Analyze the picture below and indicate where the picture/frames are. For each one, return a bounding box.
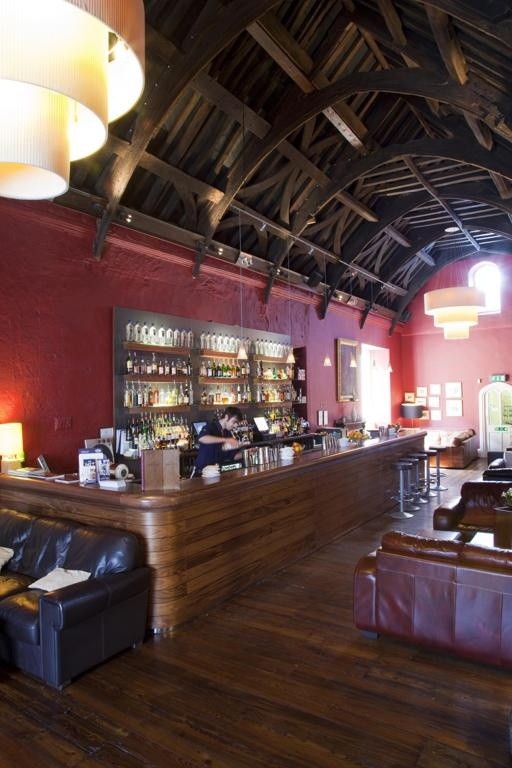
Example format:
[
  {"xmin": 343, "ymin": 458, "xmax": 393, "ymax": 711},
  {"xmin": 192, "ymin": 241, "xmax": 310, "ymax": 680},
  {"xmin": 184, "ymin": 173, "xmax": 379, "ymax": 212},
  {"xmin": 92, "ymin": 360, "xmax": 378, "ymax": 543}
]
[
  {"xmin": 336, "ymin": 339, "xmax": 361, "ymax": 403},
  {"xmin": 404, "ymin": 392, "xmax": 415, "ymax": 403}
]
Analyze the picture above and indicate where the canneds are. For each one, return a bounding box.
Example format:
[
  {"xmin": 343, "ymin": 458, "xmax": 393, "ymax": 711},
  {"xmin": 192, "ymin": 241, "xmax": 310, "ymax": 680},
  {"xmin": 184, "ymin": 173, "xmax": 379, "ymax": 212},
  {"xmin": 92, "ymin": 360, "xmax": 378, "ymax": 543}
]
[
  {"xmin": 243, "ymin": 447, "xmax": 278, "ymax": 468},
  {"xmin": 322, "ymin": 436, "xmax": 339, "ymax": 450}
]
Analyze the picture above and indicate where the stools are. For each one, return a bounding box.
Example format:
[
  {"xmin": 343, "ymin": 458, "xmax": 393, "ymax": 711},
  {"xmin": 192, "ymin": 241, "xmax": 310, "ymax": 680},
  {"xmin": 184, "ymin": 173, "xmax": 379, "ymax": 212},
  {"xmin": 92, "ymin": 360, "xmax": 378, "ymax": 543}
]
[
  {"xmin": 407, "ymin": 453, "xmax": 428, "ymax": 504},
  {"xmin": 429, "ymin": 446, "xmax": 448, "ymax": 491},
  {"xmin": 419, "ymin": 450, "xmax": 438, "ymax": 497},
  {"xmin": 399, "ymin": 458, "xmax": 420, "ymax": 512},
  {"xmin": 390, "ymin": 461, "xmax": 414, "ymax": 520}
]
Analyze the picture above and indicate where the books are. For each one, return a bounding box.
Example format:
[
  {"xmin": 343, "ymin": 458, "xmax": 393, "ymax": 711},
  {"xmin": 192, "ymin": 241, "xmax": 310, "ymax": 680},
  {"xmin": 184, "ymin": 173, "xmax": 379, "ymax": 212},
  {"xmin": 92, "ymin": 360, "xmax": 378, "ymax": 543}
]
[{"xmin": 11, "ymin": 467, "xmax": 81, "ymax": 484}]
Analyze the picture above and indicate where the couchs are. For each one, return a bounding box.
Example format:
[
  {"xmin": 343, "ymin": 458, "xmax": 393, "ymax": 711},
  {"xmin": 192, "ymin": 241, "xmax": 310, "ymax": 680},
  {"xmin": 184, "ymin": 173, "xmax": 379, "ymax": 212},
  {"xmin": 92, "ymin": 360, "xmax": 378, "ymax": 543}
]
[
  {"xmin": 401, "ymin": 428, "xmax": 480, "ymax": 469},
  {"xmin": 0, "ymin": 504, "xmax": 155, "ymax": 688},
  {"xmin": 483, "ymin": 448, "xmax": 512, "ymax": 481},
  {"xmin": 433, "ymin": 480, "xmax": 512, "ymax": 531},
  {"xmin": 355, "ymin": 530, "xmax": 512, "ymax": 671}
]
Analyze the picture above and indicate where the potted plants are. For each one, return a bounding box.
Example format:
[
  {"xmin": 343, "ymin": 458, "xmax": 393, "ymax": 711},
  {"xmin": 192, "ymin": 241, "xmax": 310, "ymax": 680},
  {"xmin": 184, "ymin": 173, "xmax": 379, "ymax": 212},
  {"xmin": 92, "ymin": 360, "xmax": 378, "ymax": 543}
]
[{"xmin": 491, "ymin": 488, "xmax": 512, "ymax": 531}]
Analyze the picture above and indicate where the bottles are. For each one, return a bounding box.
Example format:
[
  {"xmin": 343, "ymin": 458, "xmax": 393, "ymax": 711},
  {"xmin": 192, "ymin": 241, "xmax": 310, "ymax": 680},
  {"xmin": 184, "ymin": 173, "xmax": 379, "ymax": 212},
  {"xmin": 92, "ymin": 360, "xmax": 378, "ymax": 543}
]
[
  {"xmin": 222, "ymin": 383, "xmax": 252, "ymax": 403},
  {"xmin": 166, "ymin": 384, "xmax": 172, "ymax": 405},
  {"xmin": 146, "ymin": 359, "xmax": 152, "ymax": 373},
  {"xmin": 158, "ymin": 359, "xmax": 164, "ymax": 374},
  {"xmin": 223, "ymin": 333, "xmax": 230, "ymax": 351},
  {"xmin": 217, "ymin": 332, "xmax": 224, "ymax": 351},
  {"xmin": 200, "ymin": 330, "xmax": 206, "ymax": 349},
  {"xmin": 240, "ymin": 361, "xmax": 246, "ymax": 377},
  {"xmin": 138, "ymin": 384, "xmax": 143, "ymax": 406},
  {"xmin": 215, "ymin": 384, "xmax": 222, "ymax": 404},
  {"xmin": 171, "ymin": 354, "xmax": 191, "ymax": 374},
  {"xmin": 140, "ymin": 353, "xmax": 146, "ymax": 374},
  {"xmin": 164, "ymin": 358, "xmax": 169, "ymax": 374},
  {"xmin": 199, "ymin": 360, "xmax": 207, "ymax": 377},
  {"xmin": 150, "ymin": 322, "xmax": 157, "ymax": 344},
  {"xmin": 236, "ymin": 335, "xmax": 251, "ymax": 353},
  {"xmin": 260, "ymin": 338, "xmax": 264, "ymax": 354},
  {"xmin": 125, "ymin": 319, "xmax": 134, "ymax": 341},
  {"xmin": 178, "ymin": 383, "xmax": 184, "ymax": 405},
  {"xmin": 210, "ymin": 331, "xmax": 217, "ymax": 349},
  {"xmin": 180, "ymin": 327, "xmax": 188, "ymax": 347},
  {"xmin": 255, "ymin": 383, "xmax": 292, "ymax": 402},
  {"xmin": 264, "ymin": 339, "xmax": 293, "ymax": 357},
  {"xmin": 255, "ymin": 338, "xmax": 260, "ymax": 354},
  {"xmin": 207, "ymin": 360, "xmax": 212, "ymax": 376},
  {"xmin": 148, "ymin": 384, "xmax": 154, "ymax": 406},
  {"xmin": 172, "ymin": 383, "xmax": 178, "ymax": 405},
  {"xmin": 206, "ymin": 331, "xmax": 211, "ymax": 349},
  {"xmin": 133, "ymin": 351, "xmax": 139, "ymax": 373},
  {"xmin": 142, "ymin": 321, "xmax": 150, "ymax": 344},
  {"xmin": 201, "ymin": 386, "xmax": 207, "ymax": 405},
  {"xmin": 166, "ymin": 325, "xmax": 173, "ymax": 346},
  {"xmin": 152, "ymin": 352, "xmax": 157, "ymax": 373},
  {"xmin": 187, "ymin": 327, "xmax": 194, "ymax": 347},
  {"xmin": 154, "ymin": 384, "xmax": 159, "ymax": 406},
  {"xmin": 222, "ymin": 441, "xmax": 251, "ymax": 451},
  {"xmin": 126, "ymin": 412, "xmax": 194, "ymax": 450},
  {"xmin": 125, "ymin": 349, "xmax": 133, "ymax": 373},
  {"xmin": 246, "ymin": 362, "xmax": 251, "ymax": 377},
  {"xmin": 130, "ymin": 384, "xmax": 137, "ymax": 407},
  {"xmin": 143, "ymin": 384, "xmax": 148, "ymax": 407},
  {"xmin": 134, "ymin": 320, "xmax": 142, "ymax": 342},
  {"xmin": 173, "ymin": 327, "xmax": 181, "ymax": 347},
  {"xmin": 229, "ymin": 334, "xmax": 236, "ymax": 352},
  {"xmin": 184, "ymin": 383, "xmax": 189, "ymax": 404},
  {"xmin": 123, "ymin": 383, "xmax": 130, "ymax": 407},
  {"xmin": 264, "ymin": 408, "xmax": 304, "ymax": 436},
  {"xmin": 256, "ymin": 360, "xmax": 293, "ymax": 380},
  {"xmin": 159, "ymin": 387, "xmax": 166, "ymax": 404},
  {"xmin": 157, "ymin": 323, "xmax": 166, "ymax": 345},
  {"xmin": 207, "ymin": 387, "xmax": 214, "ymax": 405},
  {"xmin": 222, "ymin": 361, "xmax": 241, "ymax": 377},
  {"xmin": 215, "ymin": 408, "xmax": 225, "ymax": 418},
  {"xmin": 213, "ymin": 360, "xmax": 222, "ymax": 377}
]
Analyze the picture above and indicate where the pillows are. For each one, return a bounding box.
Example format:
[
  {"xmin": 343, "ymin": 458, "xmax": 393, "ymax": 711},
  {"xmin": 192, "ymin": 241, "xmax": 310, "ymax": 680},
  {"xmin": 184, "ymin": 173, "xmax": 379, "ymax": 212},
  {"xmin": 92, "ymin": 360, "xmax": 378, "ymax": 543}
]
[
  {"xmin": 0, "ymin": 547, "xmax": 14, "ymax": 571},
  {"xmin": 28, "ymin": 567, "xmax": 91, "ymax": 592}
]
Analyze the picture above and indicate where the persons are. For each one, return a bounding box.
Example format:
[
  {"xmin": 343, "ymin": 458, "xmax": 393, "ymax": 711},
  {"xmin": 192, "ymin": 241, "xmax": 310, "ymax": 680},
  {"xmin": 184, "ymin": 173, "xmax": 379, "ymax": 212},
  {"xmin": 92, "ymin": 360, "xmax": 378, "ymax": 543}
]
[{"xmin": 195, "ymin": 406, "xmax": 243, "ymax": 477}]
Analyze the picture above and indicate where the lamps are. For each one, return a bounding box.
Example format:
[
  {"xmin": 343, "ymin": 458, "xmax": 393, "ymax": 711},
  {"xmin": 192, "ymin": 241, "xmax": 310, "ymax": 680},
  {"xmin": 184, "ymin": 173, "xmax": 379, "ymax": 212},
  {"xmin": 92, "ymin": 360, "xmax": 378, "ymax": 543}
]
[
  {"xmin": 0, "ymin": 0, "xmax": 146, "ymax": 200},
  {"xmin": 0, "ymin": 422, "xmax": 23, "ymax": 474},
  {"xmin": 322, "ymin": 253, "xmax": 333, "ymax": 367},
  {"xmin": 349, "ymin": 274, "xmax": 357, "ymax": 368},
  {"xmin": 285, "ymin": 241, "xmax": 296, "ymax": 364},
  {"xmin": 235, "ymin": 208, "xmax": 249, "ymax": 360},
  {"xmin": 401, "ymin": 403, "xmax": 422, "ymax": 428},
  {"xmin": 424, "ymin": 227, "xmax": 486, "ymax": 340}
]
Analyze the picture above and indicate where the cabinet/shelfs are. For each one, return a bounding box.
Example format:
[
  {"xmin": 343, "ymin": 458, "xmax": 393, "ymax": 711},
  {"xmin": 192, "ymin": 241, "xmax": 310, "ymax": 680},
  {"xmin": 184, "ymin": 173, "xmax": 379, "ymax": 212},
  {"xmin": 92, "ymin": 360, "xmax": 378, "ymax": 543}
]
[{"xmin": 112, "ymin": 306, "xmax": 295, "ymax": 476}]
